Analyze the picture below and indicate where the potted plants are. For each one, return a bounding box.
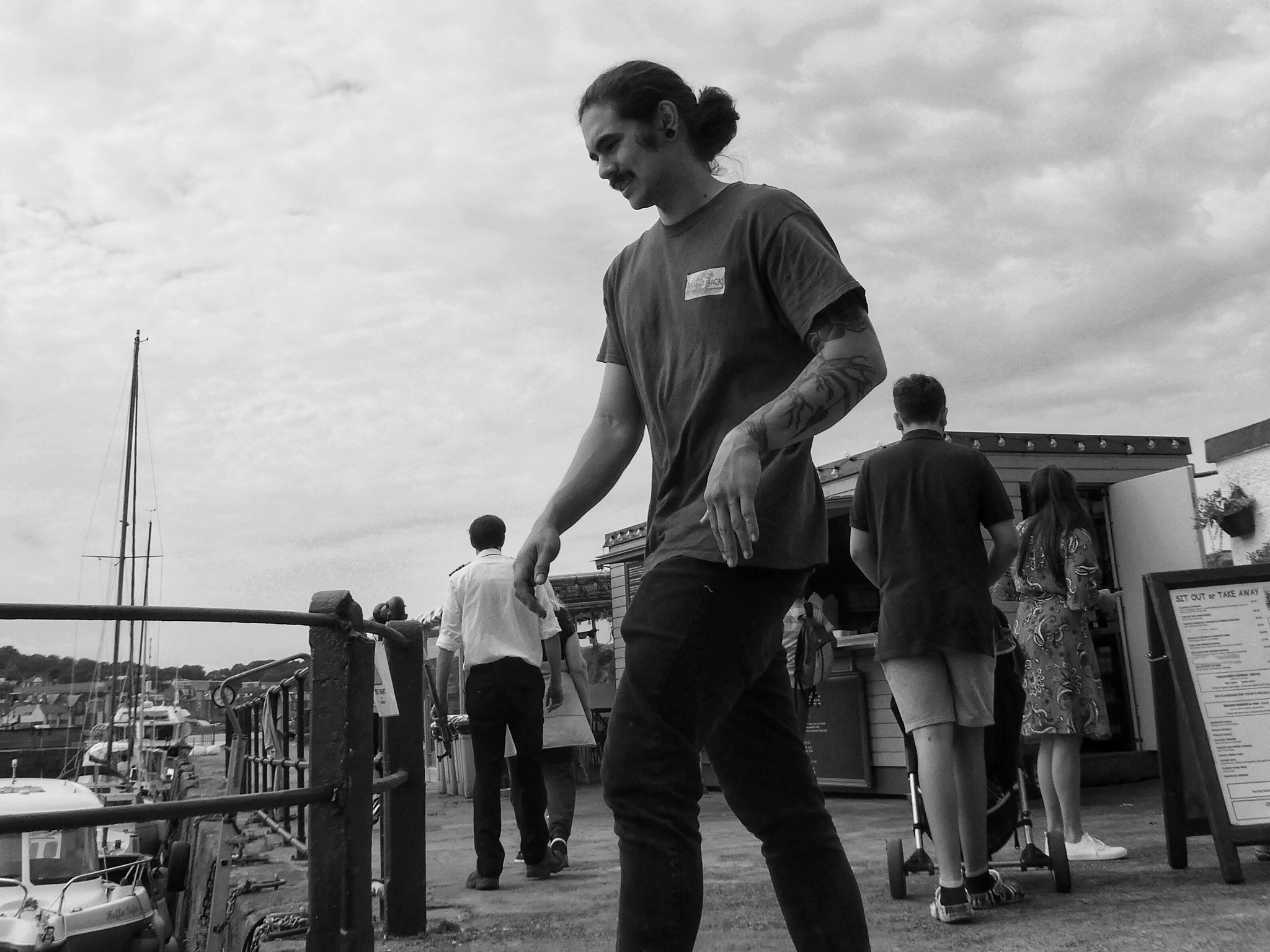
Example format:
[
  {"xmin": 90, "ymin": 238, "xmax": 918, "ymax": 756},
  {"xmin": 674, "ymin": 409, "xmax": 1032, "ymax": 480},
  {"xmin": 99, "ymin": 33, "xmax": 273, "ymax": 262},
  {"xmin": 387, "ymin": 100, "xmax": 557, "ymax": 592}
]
[{"xmin": 1193, "ymin": 484, "xmax": 1256, "ymax": 537}]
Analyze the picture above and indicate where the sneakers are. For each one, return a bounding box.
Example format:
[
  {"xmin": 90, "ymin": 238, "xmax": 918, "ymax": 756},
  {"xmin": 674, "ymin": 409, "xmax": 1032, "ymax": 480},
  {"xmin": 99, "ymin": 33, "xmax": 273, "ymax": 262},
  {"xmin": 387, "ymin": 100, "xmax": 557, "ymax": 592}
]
[
  {"xmin": 1064, "ymin": 832, "xmax": 1127, "ymax": 860},
  {"xmin": 1043, "ymin": 830, "xmax": 1050, "ymax": 857}
]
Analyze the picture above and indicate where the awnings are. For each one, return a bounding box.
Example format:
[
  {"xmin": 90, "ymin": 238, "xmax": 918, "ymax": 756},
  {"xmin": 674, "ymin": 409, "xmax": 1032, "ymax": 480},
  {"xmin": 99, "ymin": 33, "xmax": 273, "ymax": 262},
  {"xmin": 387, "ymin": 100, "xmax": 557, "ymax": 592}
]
[{"xmin": 590, "ymin": 496, "xmax": 855, "ymax": 569}]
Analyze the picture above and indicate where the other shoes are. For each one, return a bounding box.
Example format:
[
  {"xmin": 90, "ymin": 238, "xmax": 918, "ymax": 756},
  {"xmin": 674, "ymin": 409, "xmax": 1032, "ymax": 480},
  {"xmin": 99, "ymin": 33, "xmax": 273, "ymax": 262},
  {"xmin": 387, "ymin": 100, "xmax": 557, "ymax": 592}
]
[
  {"xmin": 550, "ymin": 829, "xmax": 569, "ymax": 867},
  {"xmin": 514, "ymin": 851, "xmax": 525, "ymax": 863},
  {"xmin": 527, "ymin": 846, "xmax": 553, "ymax": 878},
  {"xmin": 465, "ymin": 866, "xmax": 499, "ymax": 890}
]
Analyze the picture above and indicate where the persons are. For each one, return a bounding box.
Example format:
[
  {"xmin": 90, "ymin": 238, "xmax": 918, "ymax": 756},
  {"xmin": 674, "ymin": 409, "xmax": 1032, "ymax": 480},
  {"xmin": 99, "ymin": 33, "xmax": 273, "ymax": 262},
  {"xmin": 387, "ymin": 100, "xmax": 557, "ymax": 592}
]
[
  {"xmin": 849, "ymin": 373, "xmax": 1025, "ymax": 923},
  {"xmin": 783, "ymin": 583, "xmax": 838, "ymax": 745},
  {"xmin": 994, "ymin": 465, "xmax": 1127, "ymax": 861},
  {"xmin": 430, "ymin": 515, "xmax": 596, "ymax": 890},
  {"xmin": 511, "ymin": 61, "xmax": 890, "ymax": 952}
]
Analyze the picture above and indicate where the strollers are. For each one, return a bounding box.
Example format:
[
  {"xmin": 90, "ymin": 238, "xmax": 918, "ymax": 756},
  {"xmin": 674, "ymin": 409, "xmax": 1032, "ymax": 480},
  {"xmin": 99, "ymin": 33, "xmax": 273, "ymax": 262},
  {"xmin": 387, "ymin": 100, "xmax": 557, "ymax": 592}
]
[{"xmin": 883, "ymin": 596, "xmax": 1074, "ymax": 898}]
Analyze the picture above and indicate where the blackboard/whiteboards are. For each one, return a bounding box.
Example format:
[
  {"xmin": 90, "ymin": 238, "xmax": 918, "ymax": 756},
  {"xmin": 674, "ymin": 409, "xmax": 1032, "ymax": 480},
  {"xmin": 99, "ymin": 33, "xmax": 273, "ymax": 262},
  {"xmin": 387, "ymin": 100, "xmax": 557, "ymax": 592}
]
[{"xmin": 802, "ymin": 671, "xmax": 870, "ymax": 791}]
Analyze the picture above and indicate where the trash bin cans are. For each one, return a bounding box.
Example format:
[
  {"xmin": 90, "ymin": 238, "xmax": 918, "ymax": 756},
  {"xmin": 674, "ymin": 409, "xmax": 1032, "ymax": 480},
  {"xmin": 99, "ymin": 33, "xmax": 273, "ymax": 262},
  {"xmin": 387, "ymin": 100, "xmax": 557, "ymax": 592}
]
[{"xmin": 430, "ymin": 714, "xmax": 476, "ymax": 798}]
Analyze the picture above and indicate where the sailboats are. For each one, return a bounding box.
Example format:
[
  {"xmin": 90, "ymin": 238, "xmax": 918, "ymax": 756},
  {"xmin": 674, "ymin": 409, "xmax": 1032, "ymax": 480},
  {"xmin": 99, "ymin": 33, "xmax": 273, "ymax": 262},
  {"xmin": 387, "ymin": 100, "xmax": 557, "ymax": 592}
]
[{"xmin": 1, "ymin": 331, "xmax": 192, "ymax": 952}]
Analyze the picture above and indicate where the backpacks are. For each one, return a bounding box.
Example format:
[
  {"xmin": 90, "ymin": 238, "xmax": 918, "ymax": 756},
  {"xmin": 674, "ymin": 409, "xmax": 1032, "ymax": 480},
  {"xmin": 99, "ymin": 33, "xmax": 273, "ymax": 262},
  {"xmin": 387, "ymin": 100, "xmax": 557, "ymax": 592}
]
[{"xmin": 797, "ymin": 600, "xmax": 837, "ymax": 688}]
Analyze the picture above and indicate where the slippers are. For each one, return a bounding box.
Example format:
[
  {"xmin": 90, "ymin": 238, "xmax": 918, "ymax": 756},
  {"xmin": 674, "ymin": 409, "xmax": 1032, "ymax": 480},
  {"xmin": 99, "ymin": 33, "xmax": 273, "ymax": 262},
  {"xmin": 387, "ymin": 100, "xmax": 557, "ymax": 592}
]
[
  {"xmin": 964, "ymin": 869, "xmax": 1025, "ymax": 909},
  {"xmin": 930, "ymin": 886, "xmax": 974, "ymax": 922}
]
